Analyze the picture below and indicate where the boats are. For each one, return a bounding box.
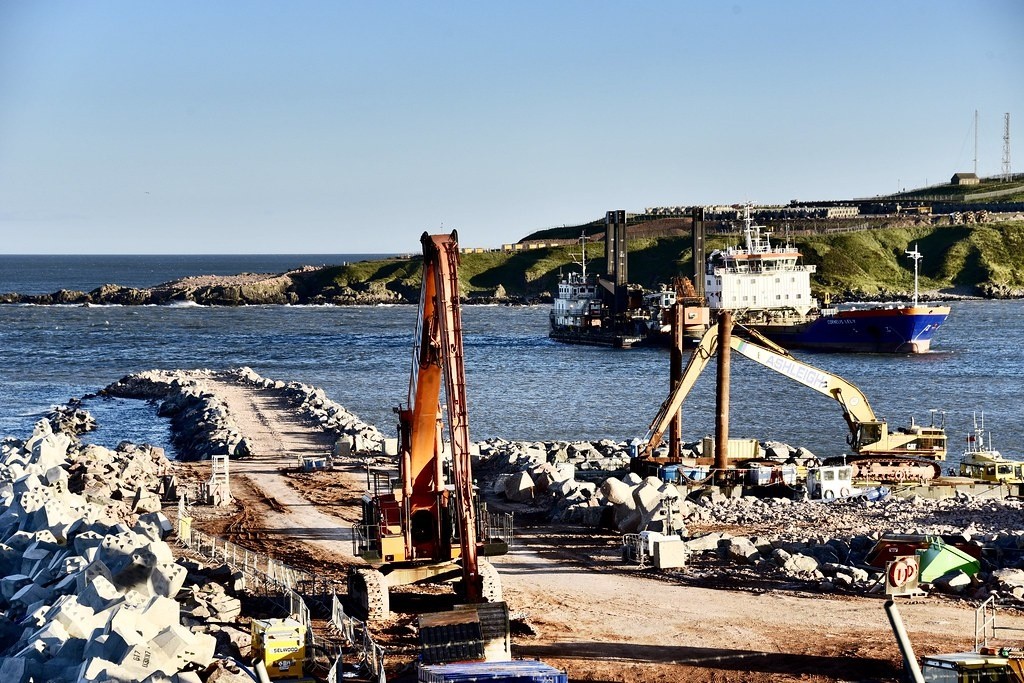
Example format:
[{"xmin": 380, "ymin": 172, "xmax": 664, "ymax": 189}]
[
  {"xmin": 704, "ymin": 206, "xmax": 951, "ymax": 355},
  {"xmin": 949, "ymin": 410, "xmax": 1024, "ymax": 486},
  {"xmin": 548, "ymin": 227, "xmax": 609, "ymax": 340}
]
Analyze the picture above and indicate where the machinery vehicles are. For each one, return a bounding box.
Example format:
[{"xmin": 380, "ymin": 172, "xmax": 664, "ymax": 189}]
[
  {"xmin": 347, "ymin": 229, "xmax": 508, "ymax": 623},
  {"xmin": 920, "ymin": 646, "xmax": 1024, "ymax": 683},
  {"xmin": 638, "ymin": 317, "xmax": 946, "ymax": 483}
]
